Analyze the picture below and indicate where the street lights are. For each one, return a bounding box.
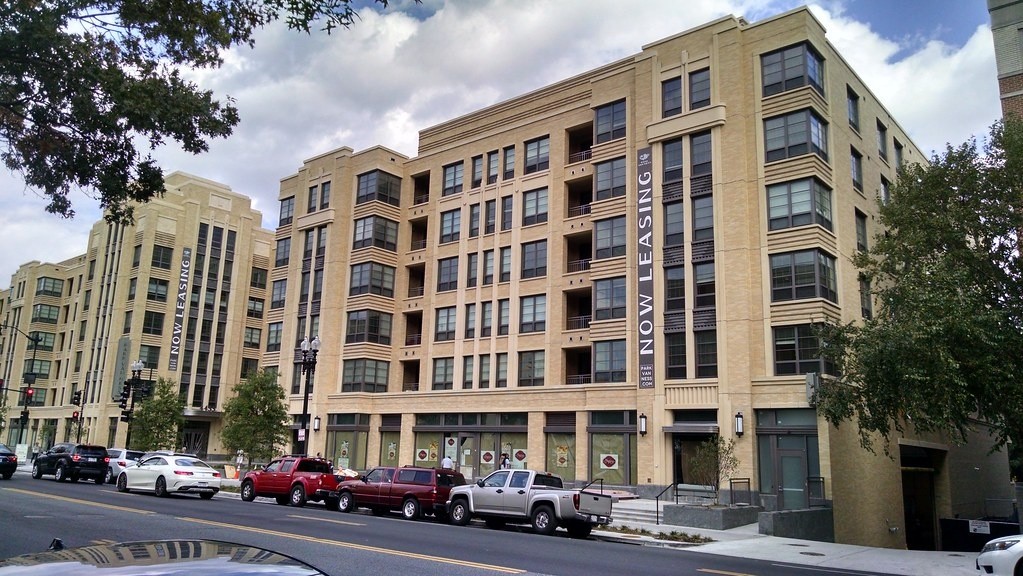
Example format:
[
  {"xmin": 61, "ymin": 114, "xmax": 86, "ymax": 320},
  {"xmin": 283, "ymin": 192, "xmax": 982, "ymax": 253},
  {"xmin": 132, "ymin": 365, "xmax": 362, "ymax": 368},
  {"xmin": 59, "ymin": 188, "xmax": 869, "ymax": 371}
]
[
  {"xmin": 297, "ymin": 336, "xmax": 321, "ymax": 458},
  {"xmin": 125, "ymin": 359, "xmax": 145, "ymax": 449}
]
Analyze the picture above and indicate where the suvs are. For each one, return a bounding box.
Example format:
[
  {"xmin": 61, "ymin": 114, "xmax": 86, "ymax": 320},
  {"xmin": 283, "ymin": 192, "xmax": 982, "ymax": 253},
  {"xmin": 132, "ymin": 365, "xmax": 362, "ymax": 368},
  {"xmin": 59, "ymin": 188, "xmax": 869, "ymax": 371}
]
[
  {"xmin": 31, "ymin": 443, "xmax": 110, "ymax": 485},
  {"xmin": 105, "ymin": 449, "xmax": 146, "ymax": 484}
]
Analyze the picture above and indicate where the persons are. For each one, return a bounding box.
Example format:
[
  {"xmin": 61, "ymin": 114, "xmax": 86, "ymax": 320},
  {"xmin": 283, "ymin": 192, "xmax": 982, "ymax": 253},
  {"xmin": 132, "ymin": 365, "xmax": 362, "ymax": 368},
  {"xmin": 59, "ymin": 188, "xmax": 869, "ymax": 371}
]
[
  {"xmin": 317, "ymin": 452, "xmax": 322, "ymax": 458},
  {"xmin": 30, "ymin": 442, "xmax": 40, "ymax": 463},
  {"xmin": 500, "ymin": 452, "xmax": 511, "ymax": 469},
  {"xmin": 236, "ymin": 449, "xmax": 244, "ymax": 472},
  {"xmin": 442, "ymin": 456, "xmax": 453, "ymax": 470},
  {"xmin": 338, "ymin": 467, "xmax": 347, "ymax": 476}
]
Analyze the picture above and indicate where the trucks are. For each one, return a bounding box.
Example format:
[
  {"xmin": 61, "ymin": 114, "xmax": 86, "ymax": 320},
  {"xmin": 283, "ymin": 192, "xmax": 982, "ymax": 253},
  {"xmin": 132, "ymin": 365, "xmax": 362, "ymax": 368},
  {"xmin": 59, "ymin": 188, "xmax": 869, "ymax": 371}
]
[{"xmin": 241, "ymin": 454, "xmax": 360, "ymax": 507}]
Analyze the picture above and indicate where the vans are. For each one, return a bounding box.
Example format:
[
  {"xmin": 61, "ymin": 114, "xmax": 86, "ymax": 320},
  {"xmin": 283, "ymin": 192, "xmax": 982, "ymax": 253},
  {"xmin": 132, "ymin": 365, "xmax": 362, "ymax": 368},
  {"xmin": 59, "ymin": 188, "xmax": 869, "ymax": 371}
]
[{"xmin": 336, "ymin": 465, "xmax": 467, "ymax": 521}]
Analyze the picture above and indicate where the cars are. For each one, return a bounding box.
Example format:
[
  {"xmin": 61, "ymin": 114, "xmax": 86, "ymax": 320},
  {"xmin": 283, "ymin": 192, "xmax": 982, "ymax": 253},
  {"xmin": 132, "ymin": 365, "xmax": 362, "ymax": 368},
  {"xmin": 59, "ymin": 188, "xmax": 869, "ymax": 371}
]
[
  {"xmin": 0, "ymin": 443, "xmax": 18, "ymax": 480},
  {"xmin": 976, "ymin": 534, "xmax": 1023, "ymax": 576},
  {"xmin": 116, "ymin": 450, "xmax": 222, "ymax": 499}
]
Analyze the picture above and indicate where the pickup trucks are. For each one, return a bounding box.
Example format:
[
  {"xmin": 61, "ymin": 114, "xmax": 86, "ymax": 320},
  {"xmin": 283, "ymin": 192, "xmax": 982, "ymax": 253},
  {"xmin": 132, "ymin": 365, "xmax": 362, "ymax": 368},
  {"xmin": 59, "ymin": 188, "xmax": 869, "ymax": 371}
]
[{"xmin": 445, "ymin": 468, "xmax": 614, "ymax": 541}]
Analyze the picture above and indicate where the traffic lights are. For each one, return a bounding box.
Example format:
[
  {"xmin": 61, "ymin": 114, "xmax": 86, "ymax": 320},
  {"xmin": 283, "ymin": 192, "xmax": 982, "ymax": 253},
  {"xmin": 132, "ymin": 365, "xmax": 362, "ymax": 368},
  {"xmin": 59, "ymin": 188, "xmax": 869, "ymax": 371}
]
[
  {"xmin": 120, "ymin": 410, "xmax": 130, "ymax": 422},
  {"xmin": 26, "ymin": 388, "xmax": 34, "ymax": 404},
  {"xmin": 73, "ymin": 412, "xmax": 79, "ymax": 422},
  {"xmin": 134, "ymin": 387, "xmax": 148, "ymax": 399},
  {"xmin": 24, "ymin": 372, "xmax": 37, "ymax": 384},
  {"xmin": 119, "ymin": 380, "xmax": 130, "ymax": 410},
  {"xmin": 20, "ymin": 410, "xmax": 29, "ymax": 425},
  {"xmin": 73, "ymin": 390, "xmax": 81, "ymax": 406}
]
